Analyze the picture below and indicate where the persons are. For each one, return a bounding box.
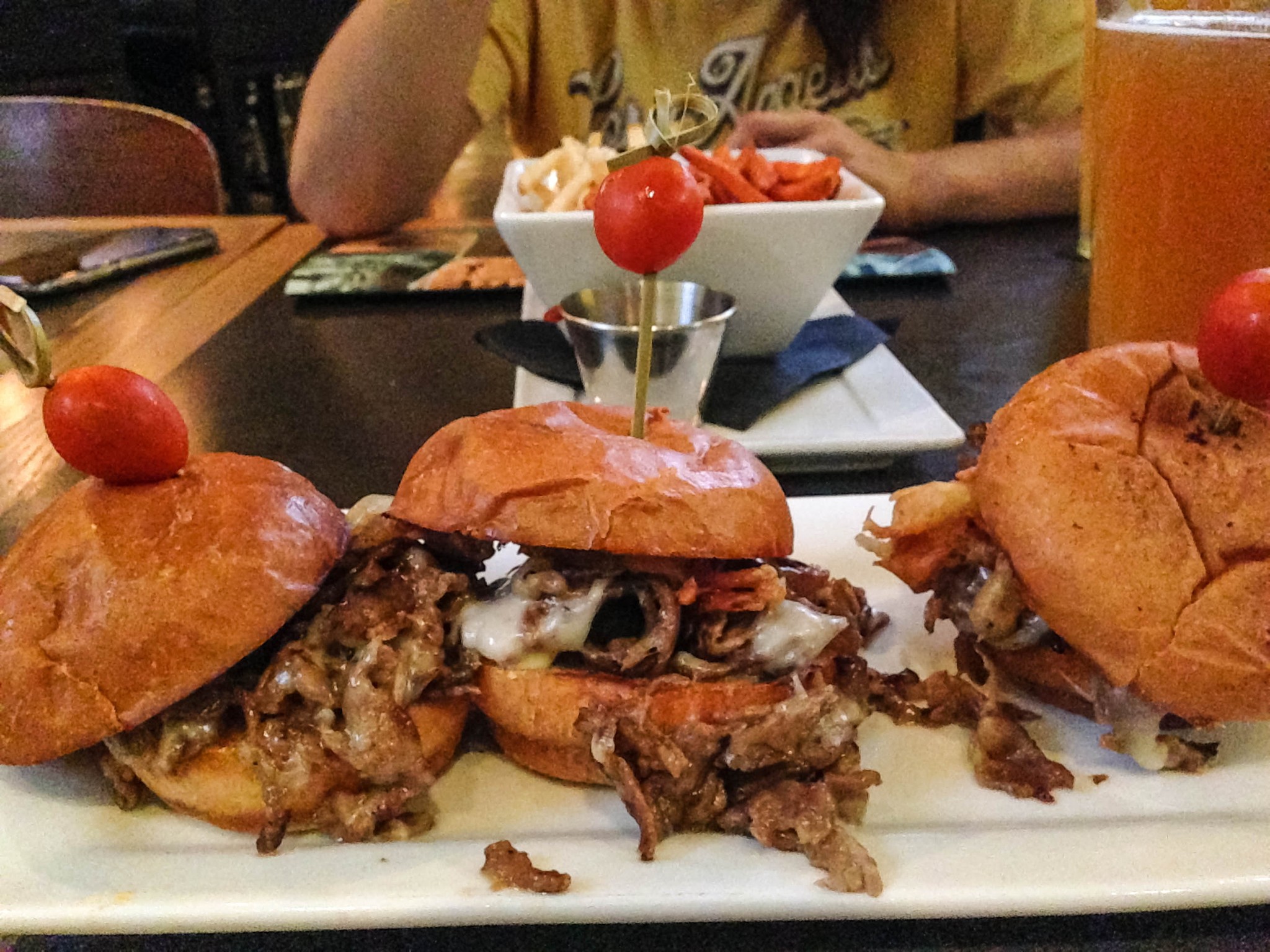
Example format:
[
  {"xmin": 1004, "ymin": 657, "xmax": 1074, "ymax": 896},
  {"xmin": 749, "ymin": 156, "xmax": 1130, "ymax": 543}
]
[{"xmin": 289, "ymin": 0, "xmax": 1113, "ymax": 233}]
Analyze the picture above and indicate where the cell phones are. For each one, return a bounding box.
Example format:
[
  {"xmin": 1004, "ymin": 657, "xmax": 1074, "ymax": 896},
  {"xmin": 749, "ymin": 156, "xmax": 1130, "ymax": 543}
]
[{"xmin": 0, "ymin": 224, "xmax": 217, "ymax": 300}]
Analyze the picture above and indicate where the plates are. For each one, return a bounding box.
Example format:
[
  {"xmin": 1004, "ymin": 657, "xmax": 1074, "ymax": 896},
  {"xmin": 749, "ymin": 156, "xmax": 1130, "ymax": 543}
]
[
  {"xmin": 513, "ymin": 263, "xmax": 969, "ymax": 490},
  {"xmin": 1, "ymin": 495, "xmax": 1270, "ymax": 940}
]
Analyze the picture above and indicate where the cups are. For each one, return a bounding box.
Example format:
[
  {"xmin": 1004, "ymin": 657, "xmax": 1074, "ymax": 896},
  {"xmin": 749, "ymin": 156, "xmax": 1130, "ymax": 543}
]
[
  {"xmin": 564, "ymin": 279, "xmax": 738, "ymax": 440},
  {"xmin": 1081, "ymin": 0, "xmax": 1270, "ymax": 429}
]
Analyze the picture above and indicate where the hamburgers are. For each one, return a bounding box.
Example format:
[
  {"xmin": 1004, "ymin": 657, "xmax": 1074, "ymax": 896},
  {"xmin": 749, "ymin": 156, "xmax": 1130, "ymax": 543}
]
[
  {"xmin": 390, "ymin": 151, "xmax": 861, "ymax": 793},
  {"xmin": 868, "ymin": 267, "xmax": 1270, "ymax": 752},
  {"xmin": 0, "ymin": 361, "xmax": 467, "ymax": 837}
]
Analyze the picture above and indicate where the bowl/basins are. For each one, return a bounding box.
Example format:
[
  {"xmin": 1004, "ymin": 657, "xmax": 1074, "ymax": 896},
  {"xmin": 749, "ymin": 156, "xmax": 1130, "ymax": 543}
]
[{"xmin": 493, "ymin": 124, "xmax": 888, "ymax": 363}]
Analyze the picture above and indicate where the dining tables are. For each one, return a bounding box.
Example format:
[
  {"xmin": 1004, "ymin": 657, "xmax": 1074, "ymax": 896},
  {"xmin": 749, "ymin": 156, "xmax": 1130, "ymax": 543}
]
[{"xmin": 0, "ymin": 191, "xmax": 1270, "ymax": 951}]
